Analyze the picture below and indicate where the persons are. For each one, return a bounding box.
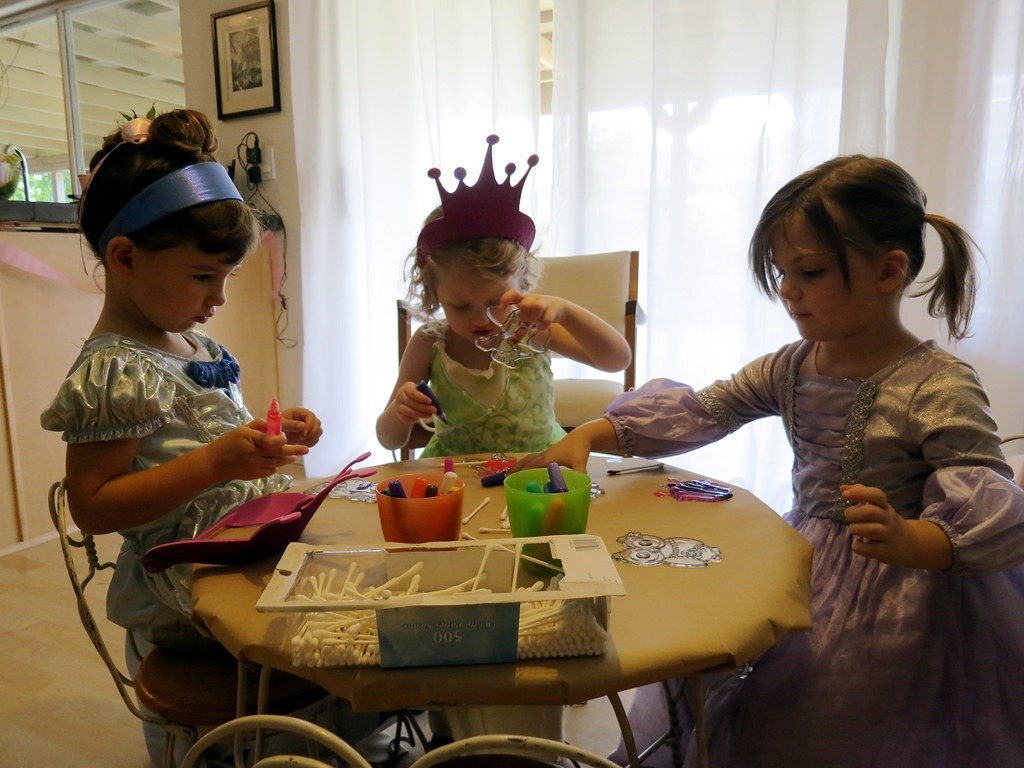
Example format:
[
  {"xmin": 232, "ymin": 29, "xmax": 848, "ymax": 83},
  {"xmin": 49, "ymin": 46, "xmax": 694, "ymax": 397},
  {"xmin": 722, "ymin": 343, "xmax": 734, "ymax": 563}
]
[
  {"xmin": 39, "ymin": 103, "xmax": 334, "ymax": 768},
  {"xmin": 376, "ymin": 201, "xmax": 633, "ymax": 459},
  {"xmin": 512, "ymin": 154, "xmax": 1024, "ymax": 768}
]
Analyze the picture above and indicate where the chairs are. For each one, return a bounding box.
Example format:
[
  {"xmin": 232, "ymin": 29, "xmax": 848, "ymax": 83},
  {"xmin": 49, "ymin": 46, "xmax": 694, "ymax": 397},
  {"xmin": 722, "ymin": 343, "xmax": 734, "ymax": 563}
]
[
  {"xmin": 177, "ymin": 713, "xmax": 370, "ymax": 768},
  {"xmin": 40, "ymin": 469, "xmax": 344, "ymax": 768},
  {"xmin": 394, "ymin": 245, "xmax": 641, "ymax": 465},
  {"xmin": 410, "ymin": 735, "xmax": 621, "ymax": 768}
]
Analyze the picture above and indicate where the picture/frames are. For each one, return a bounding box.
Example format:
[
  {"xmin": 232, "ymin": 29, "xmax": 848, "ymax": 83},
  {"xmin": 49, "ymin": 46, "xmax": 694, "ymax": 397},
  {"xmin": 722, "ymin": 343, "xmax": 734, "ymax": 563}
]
[{"xmin": 210, "ymin": 2, "xmax": 281, "ymax": 123}]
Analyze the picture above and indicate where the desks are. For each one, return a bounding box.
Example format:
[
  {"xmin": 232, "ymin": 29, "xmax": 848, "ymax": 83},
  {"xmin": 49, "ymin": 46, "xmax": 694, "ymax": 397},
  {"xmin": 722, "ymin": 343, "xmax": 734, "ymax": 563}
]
[{"xmin": 185, "ymin": 451, "xmax": 812, "ymax": 768}]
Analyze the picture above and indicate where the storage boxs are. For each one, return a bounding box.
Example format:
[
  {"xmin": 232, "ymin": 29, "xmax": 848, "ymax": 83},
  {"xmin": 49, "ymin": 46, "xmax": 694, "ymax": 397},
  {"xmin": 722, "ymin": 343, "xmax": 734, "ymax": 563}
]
[{"xmin": 257, "ymin": 541, "xmax": 622, "ymax": 665}]
